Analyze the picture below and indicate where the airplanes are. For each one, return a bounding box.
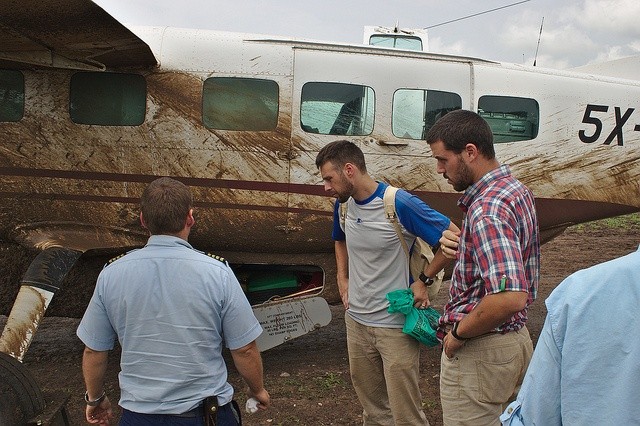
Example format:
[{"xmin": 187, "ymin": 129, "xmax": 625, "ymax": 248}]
[{"xmin": 1, "ymin": 0, "xmax": 640, "ymax": 363}]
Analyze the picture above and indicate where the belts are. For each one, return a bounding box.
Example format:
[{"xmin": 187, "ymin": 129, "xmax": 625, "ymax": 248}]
[{"xmin": 173, "ymin": 409, "xmax": 205, "ymax": 418}]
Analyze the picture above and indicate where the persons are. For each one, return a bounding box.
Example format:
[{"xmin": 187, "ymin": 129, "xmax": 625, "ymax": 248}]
[
  {"xmin": 316, "ymin": 140, "xmax": 461, "ymax": 426},
  {"xmin": 427, "ymin": 109, "xmax": 541, "ymax": 426},
  {"xmin": 499, "ymin": 245, "xmax": 640, "ymax": 426},
  {"xmin": 81, "ymin": 175, "xmax": 270, "ymax": 426}
]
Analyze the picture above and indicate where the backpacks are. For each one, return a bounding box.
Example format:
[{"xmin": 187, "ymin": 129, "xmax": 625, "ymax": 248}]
[{"xmin": 341, "ymin": 186, "xmax": 444, "ymax": 301}]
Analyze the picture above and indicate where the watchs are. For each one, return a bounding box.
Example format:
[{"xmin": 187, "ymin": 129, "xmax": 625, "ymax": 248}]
[
  {"xmin": 451, "ymin": 320, "xmax": 465, "ymax": 341},
  {"xmin": 419, "ymin": 274, "xmax": 434, "ymax": 286},
  {"xmin": 84, "ymin": 390, "xmax": 106, "ymax": 406}
]
